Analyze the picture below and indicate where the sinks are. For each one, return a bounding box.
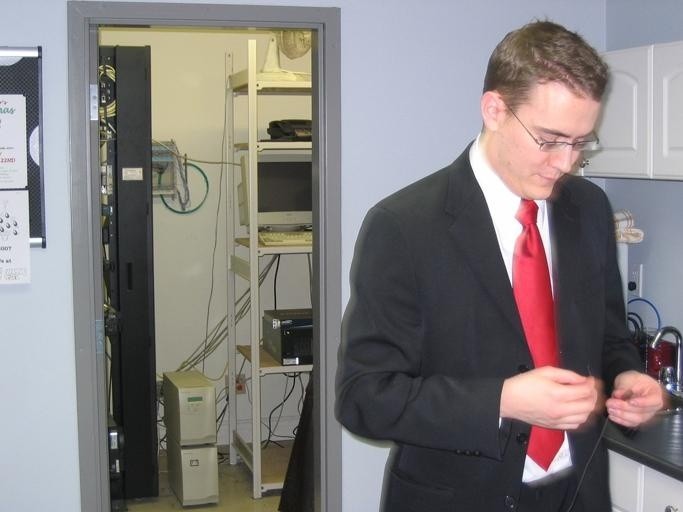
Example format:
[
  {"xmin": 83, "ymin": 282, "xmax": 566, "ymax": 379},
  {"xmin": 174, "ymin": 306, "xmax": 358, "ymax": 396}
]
[{"xmin": 653, "ymin": 390, "xmax": 683, "ymax": 416}]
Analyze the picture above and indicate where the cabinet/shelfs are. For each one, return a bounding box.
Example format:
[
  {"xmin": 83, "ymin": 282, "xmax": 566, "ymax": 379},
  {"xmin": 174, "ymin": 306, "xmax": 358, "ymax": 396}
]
[
  {"xmin": 224, "ymin": 38, "xmax": 314, "ymax": 499},
  {"xmin": 607, "ymin": 448, "xmax": 683, "ymax": 512},
  {"xmin": 572, "ymin": 38, "xmax": 683, "ymax": 183},
  {"xmin": 93, "ymin": 47, "xmax": 157, "ymax": 502}
]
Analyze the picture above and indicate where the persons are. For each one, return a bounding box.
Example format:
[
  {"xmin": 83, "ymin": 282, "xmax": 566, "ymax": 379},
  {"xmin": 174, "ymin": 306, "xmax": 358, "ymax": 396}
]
[{"xmin": 331, "ymin": 11, "xmax": 665, "ymax": 511}]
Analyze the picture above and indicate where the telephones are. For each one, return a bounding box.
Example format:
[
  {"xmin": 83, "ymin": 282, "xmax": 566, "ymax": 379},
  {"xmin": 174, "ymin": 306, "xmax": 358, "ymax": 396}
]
[{"xmin": 267, "ymin": 120, "xmax": 312, "ymax": 141}]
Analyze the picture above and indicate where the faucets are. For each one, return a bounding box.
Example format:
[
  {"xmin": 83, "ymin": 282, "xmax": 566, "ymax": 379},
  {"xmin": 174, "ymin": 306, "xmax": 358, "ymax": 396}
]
[{"xmin": 649, "ymin": 326, "xmax": 683, "ymax": 392}]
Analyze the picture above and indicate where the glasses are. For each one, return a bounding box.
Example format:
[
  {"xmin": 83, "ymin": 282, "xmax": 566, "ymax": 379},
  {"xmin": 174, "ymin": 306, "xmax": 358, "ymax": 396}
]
[{"xmin": 500, "ymin": 97, "xmax": 599, "ymax": 152}]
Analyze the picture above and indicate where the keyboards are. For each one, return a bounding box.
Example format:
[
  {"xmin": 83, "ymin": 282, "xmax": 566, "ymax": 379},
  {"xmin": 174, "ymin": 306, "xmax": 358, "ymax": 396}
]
[{"xmin": 257, "ymin": 231, "xmax": 313, "ymax": 245}]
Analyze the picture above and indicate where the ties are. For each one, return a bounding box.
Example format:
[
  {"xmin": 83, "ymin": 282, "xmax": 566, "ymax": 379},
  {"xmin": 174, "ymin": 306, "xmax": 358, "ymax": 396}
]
[{"xmin": 512, "ymin": 199, "xmax": 564, "ymax": 472}]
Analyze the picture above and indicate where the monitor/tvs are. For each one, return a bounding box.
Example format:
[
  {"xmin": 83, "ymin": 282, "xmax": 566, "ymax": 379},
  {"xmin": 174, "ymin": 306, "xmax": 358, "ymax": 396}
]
[{"xmin": 237, "ymin": 150, "xmax": 314, "ymax": 233}]
